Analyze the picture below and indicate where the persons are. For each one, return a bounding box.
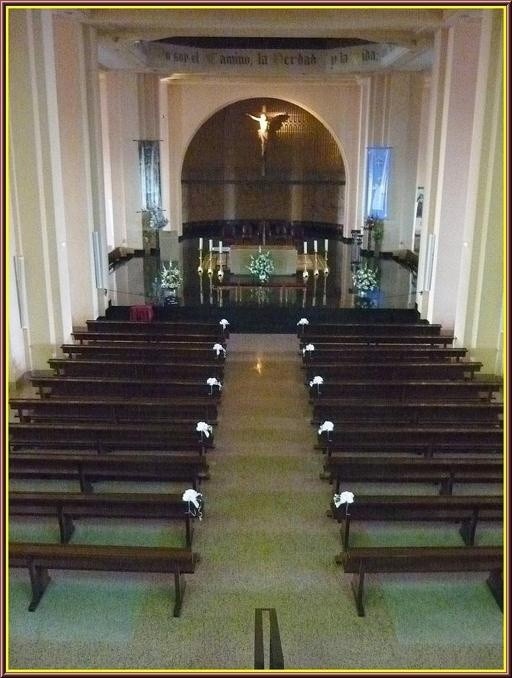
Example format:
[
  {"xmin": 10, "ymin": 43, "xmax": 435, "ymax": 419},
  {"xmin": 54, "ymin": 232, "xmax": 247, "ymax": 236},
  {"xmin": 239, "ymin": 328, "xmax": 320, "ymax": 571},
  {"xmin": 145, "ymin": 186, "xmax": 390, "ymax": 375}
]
[{"xmin": 248, "ymin": 112, "xmax": 269, "ymax": 160}]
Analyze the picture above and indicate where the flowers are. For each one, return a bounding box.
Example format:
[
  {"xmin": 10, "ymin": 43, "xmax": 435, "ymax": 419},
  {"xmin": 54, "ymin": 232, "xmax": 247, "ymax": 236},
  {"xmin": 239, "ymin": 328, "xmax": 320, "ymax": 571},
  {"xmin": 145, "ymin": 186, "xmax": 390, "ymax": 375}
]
[
  {"xmin": 153, "ymin": 257, "xmax": 185, "ymax": 291},
  {"xmin": 248, "ymin": 286, "xmax": 270, "ymax": 306},
  {"xmin": 350, "ymin": 260, "xmax": 380, "ymax": 297},
  {"xmin": 242, "ymin": 243, "xmax": 276, "ymax": 283}
]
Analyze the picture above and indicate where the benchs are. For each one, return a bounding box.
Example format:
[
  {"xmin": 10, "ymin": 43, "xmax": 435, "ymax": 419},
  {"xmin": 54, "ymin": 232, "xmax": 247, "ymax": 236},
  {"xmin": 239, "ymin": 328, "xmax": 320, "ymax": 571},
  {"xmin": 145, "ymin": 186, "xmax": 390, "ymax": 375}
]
[
  {"xmin": 292, "ymin": 308, "xmax": 506, "ymax": 622},
  {"xmin": 6, "ymin": 302, "xmax": 234, "ymax": 623}
]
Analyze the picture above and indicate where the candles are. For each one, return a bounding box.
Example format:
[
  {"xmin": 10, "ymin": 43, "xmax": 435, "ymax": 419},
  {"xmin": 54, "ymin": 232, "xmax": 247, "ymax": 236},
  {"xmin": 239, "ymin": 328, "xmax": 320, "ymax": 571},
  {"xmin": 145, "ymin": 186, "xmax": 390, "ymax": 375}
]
[
  {"xmin": 219, "ymin": 297, "xmax": 224, "ymax": 309},
  {"xmin": 322, "ymin": 294, "xmax": 327, "ymax": 306},
  {"xmin": 311, "ymin": 296, "xmax": 317, "ymax": 307},
  {"xmin": 208, "ymin": 238, "xmax": 214, "ymax": 252},
  {"xmin": 198, "ymin": 237, "xmax": 204, "ymax": 250},
  {"xmin": 209, "ymin": 296, "xmax": 214, "ymax": 305},
  {"xmin": 313, "ymin": 239, "xmax": 318, "ymax": 252},
  {"xmin": 200, "ymin": 296, "xmax": 204, "ymax": 305},
  {"xmin": 219, "ymin": 240, "xmax": 223, "ymax": 254},
  {"xmin": 324, "ymin": 238, "xmax": 328, "ymax": 252},
  {"xmin": 303, "ymin": 241, "xmax": 308, "ymax": 255}
]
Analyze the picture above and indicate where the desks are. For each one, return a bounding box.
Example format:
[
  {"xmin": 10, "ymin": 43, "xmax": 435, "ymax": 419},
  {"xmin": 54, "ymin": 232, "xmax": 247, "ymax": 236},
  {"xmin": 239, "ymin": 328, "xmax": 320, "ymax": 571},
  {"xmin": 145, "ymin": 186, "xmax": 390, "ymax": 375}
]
[{"xmin": 214, "ymin": 272, "xmax": 310, "ymax": 312}]
